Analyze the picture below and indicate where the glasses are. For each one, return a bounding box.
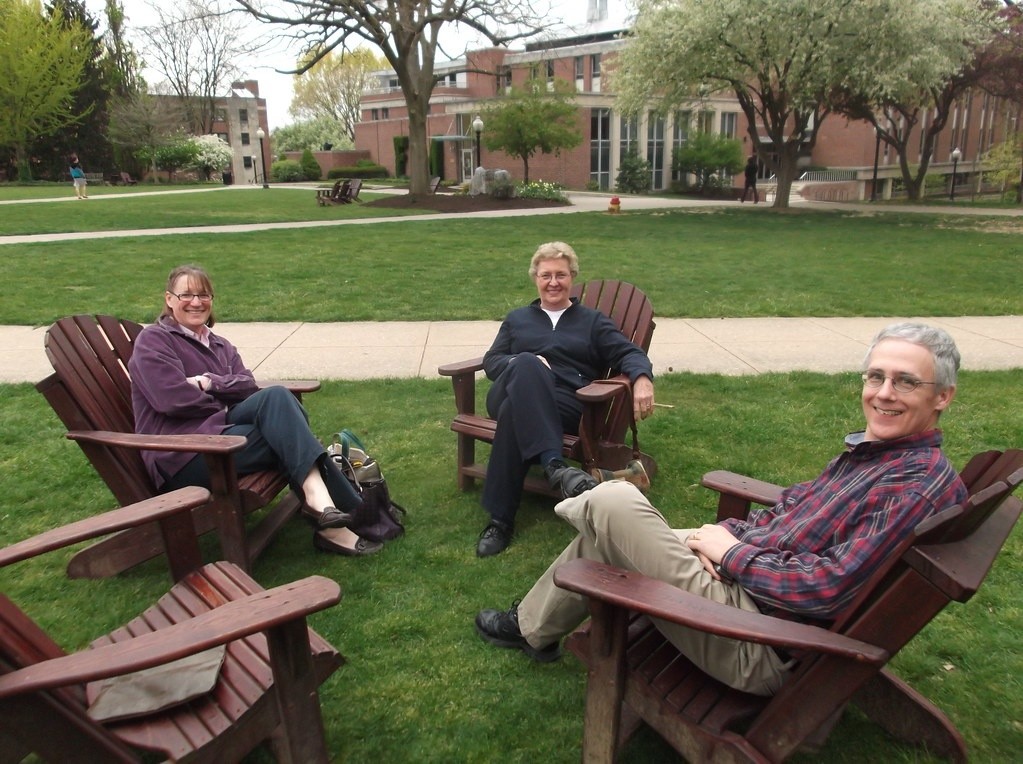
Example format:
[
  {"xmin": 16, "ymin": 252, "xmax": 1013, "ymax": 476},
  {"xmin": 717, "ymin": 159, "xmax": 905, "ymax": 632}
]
[
  {"xmin": 166, "ymin": 288, "xmax": 214, "ymax": 302},
  {"xmin": 534, "ymin": 272, "xmax": 572, "ymax": 282},
  {"xmin": 858, "ymin": 369, "xmax": 937, "ymax": 393}
]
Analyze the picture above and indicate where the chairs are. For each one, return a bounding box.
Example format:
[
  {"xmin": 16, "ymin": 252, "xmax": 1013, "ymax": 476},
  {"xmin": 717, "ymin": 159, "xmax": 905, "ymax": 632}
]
[
  {"xmin": 431, "ymin": 176, "xmax": 441, "ymax": 192},
  {"xmin": 438, "ymin": 280, "xmax": 655, "ymax": 491},
  {"xmin": 36, "ymin": 316, "xmax": 321, "ymax": 575},
  {"xmin": 552, "ymin": 446, "xmax": 1023, "ymax": 764},
  {"xmin": 120, "ymin": 172, "xmax": 137, "ymax": 187},
  {"xmin": 315, "ymin": 178, "xmax": 363, "ymax": 206},
  {"xmin": 0, "ymin": 485, "xmax": 345, "ymax": 764}
]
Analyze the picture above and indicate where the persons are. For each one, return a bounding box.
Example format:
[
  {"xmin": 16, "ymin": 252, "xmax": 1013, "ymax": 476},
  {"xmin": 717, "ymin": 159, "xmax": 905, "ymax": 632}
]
[
  {"xmin": 128, "ymin": 266, "xmax": 383, "ymax": 556},
  {"xmin": 476, "ymin": 242, "xmax": 654, "ymax": 558},
  {"xmin": 473, "ymin": 324, "xmax": 968, "ymax": 698},
  {"xmin": 70, "ymin": 153, "xmax": 88, "ymax": 199},
  {"xmin": 741, "ymin": 157, "xmax": 759, "ymax": 204}
]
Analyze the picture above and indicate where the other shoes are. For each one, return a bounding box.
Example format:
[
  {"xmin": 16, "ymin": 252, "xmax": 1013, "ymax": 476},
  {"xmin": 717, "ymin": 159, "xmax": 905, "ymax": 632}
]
[
  {"xmin": 299, "ymin": 500, "xmax": 353, "ymax": 530},
  {"xmin": 312, "ymin": 530, "xmax": 384, "ymax": 557}
]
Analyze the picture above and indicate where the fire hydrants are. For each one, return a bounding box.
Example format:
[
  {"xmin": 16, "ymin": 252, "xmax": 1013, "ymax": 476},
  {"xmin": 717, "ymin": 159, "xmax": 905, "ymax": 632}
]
[{"xmin": 608, "ymin": 195, "xmax": 620, "ymax": 214}]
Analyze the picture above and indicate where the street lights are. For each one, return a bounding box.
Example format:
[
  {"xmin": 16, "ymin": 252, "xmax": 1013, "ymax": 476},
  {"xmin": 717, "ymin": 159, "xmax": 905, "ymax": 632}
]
[
  {"xmin": 949, "ymin": 148, "xmax": 961, "ymax": 199},
  {"xmin": 472, "ymin": 115, "xmax": 484, "ymax": 167},
  {"xmin": 250, "ymin": 152, "xmax": 258, "ymax": 184},
  {"xmin": 871, "ymin": 123, "xmax": 884, "ymax": 202},
  {"xmin": 256, "ymin": 127, "xmax": 270, "ymax": 189}
]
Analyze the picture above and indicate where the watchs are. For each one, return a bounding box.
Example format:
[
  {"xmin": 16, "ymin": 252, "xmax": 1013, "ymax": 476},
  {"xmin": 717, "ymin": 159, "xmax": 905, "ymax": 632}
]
[{"xmin": 195, "ymin": 375, "xmax": 205, "ymax": 392}]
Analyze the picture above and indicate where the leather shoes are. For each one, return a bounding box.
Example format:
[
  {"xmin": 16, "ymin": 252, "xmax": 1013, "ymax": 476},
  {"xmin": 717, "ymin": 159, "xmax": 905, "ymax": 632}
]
[
  {"xmin": 476, "ymin": 518, "xmax": 512, "ymax": 558},
  {"xmin": 542, "ymin": 457, "xmax": 568, "ymax": 492},
  {"xmin": 558, "ymin": 467, "xmax": 600, "ymax": 502},
  {"xmin": 474, "ymin": 598, "xmax": 560, "ymax": 663}
]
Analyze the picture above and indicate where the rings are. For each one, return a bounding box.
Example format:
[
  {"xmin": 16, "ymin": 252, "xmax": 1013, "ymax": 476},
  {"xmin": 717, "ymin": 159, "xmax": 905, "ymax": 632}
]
[
  {"xmin": 693, "ymin": 531, "xmax": 697, "ymax": 540},
  {"xmin": 646, "ymin": 405, "xmax": 650, "ymax": 408}
]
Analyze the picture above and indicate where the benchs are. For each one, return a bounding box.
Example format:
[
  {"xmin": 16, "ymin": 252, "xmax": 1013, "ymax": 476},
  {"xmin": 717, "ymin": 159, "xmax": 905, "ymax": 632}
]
[{"xmin": 85, "ymin": 172, "xmax": 104, "ymax": 184}]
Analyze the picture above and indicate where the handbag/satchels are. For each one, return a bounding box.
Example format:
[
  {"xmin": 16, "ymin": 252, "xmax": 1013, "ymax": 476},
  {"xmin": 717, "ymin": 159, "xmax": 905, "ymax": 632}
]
[
  {"xmin": 323, "ymin": 429, "xmax": 385, "ymax": 485},
  {"xmin": 577, "ymin": 379, "xmax": 651, "ymax": 497},
  {"xmin": 324, "ymin": 453, "xmax": 407, "ymax": 542}
]
